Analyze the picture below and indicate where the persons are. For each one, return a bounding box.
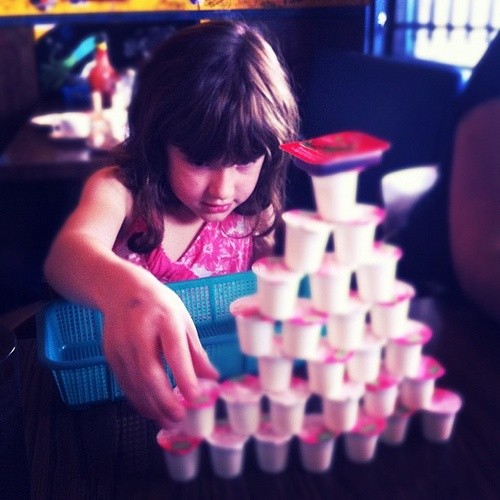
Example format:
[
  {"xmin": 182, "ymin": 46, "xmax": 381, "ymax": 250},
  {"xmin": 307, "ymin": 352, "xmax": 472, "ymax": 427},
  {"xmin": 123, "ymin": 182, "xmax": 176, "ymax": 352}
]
[
  {"xmin": 44, "ymin": 20, "xmax": 303, "ymax": 427},
  {"xmin": 389, "ymin": 30, "xmax": 499, "ymax": 353}
]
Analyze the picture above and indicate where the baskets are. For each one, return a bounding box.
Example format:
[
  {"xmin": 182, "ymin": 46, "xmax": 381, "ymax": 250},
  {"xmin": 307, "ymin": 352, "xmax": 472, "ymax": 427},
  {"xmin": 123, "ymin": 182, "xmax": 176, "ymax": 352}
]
[{"xmin": 41, "ymin": 270, "xmax": 326, "ymax": 408}]
